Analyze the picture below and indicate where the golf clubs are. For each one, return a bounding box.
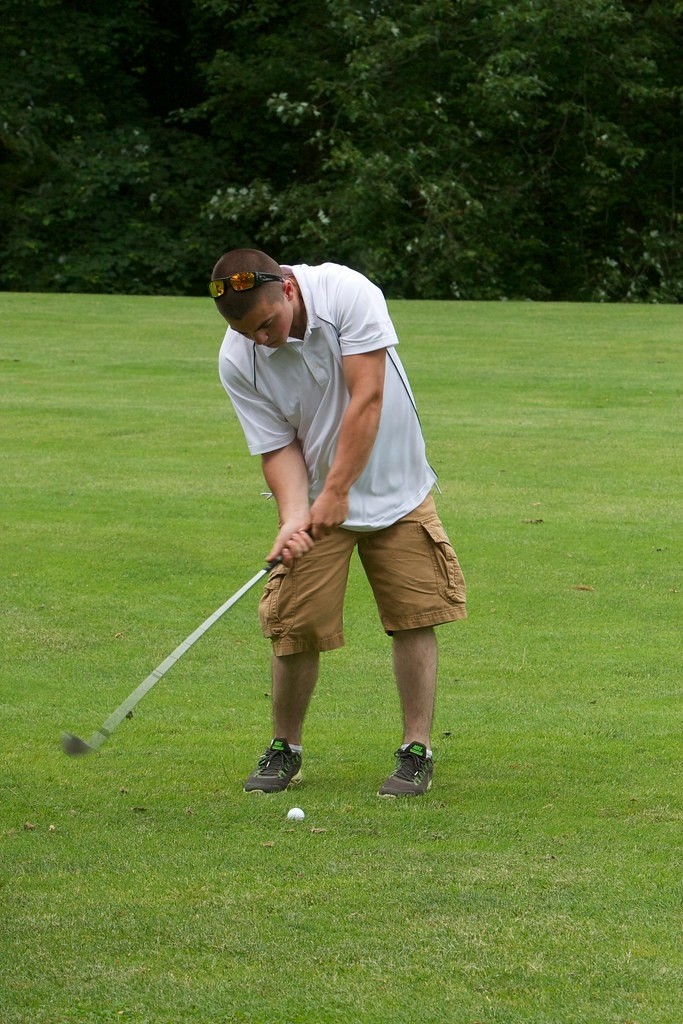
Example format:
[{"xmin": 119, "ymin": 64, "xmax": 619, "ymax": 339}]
[{"xmin": 61, "ymin": 527, "xmax": 312, "ymax": 757}]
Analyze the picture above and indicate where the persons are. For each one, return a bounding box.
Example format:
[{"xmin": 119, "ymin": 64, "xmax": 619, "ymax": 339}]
[{"xmin": 208, "ymin": 249, "xmax": 467, "ymax": 799}]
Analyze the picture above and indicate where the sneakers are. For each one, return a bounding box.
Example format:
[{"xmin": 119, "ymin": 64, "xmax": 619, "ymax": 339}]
[
  {"xmin": 377, "ymin": 741, "xmax": 433, "ymax": 798},
  {"xmin": 243, "ymin": 737, "xmax": 302, "ymax": 794}
]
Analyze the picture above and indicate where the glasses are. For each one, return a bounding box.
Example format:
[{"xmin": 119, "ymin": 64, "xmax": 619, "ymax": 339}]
[{"xmin": 208, "ymin": 271, "xmax": 284, "ymax": 299}]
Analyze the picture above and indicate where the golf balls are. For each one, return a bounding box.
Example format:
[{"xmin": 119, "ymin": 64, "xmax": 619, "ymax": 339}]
[{"xmin": 287, "ymin": 807, "xmax": 306, "ymax": 822}]
